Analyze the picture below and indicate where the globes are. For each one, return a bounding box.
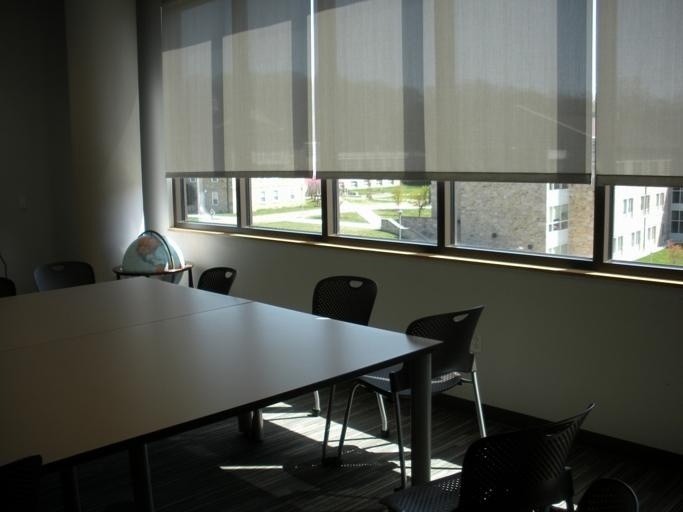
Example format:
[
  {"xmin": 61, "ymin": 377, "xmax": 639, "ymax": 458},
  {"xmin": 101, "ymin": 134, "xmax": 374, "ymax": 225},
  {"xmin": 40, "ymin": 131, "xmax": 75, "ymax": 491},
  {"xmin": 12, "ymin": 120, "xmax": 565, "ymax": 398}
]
[{"xmin": 112, "ymin": 230, "xmax": 194, "ymax": 288}]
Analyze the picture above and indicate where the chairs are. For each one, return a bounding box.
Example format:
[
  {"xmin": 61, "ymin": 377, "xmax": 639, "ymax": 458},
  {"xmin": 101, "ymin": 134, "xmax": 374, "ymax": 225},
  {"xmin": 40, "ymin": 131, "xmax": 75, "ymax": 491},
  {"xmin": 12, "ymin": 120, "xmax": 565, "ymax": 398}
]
[
  {"xmin": 197, "ymin": 267, "xmax": 236, "ymax": 295},
  {"xmin": 1, "ymin": 260, "xmax": 95, "ymax": 298},
  {"xmin": 339, "ymin": 306, "xmax": 486, "ymax": 492},
  {"xmin": 312, "ymin": 275, "xmax": 377, "ymax": 325},
  {"xmin": 575, "ymin": 477, "xmax": 639, "ymax": 511},
  {"xmin": 379, "ymin": 403, "xmax": 596, "ymax": 512}
]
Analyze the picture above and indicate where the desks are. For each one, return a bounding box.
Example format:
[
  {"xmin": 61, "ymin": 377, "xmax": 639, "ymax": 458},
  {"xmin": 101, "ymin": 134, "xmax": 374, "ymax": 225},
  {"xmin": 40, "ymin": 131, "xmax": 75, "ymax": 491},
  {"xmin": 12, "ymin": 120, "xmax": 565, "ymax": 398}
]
[{"xmin": 0, "ymin": 274, "xmax": 444, "ymax": 511}]
